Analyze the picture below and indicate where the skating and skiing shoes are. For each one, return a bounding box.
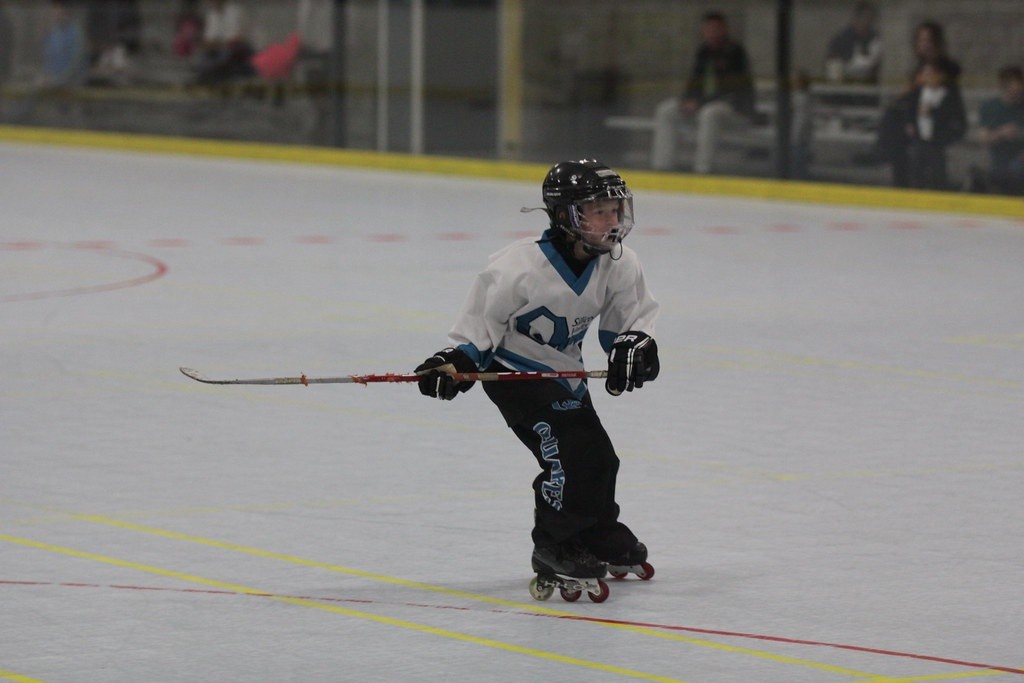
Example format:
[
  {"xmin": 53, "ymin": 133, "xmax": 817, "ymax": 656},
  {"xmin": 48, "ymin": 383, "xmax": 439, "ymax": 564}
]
[
  {"xmin": 598, "ymin": 541, "xmax": 655, "ymax": 580},
  {"xmin": 528, "ymin": 545, "xmax": 610, "ymax": 604}
]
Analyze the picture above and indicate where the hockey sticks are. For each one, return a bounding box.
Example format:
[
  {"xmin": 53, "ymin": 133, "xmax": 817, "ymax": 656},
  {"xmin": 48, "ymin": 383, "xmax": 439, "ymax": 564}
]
[{"xmin": 179, "ymin": 367, "xmax": 614, "ymax": 385}]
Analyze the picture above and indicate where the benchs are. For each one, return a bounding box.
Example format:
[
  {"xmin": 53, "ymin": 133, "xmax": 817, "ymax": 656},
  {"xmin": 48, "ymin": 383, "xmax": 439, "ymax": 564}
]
[{"xmin": 602, "ymin": 78, "xmax": 1002, "ymax": 189}]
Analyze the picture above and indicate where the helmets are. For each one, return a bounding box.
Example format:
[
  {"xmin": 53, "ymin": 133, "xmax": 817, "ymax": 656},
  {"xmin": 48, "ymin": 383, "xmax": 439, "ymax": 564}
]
[{"xmin": 542, "ymin": 159, "xmax": 632, "ymax": 259}]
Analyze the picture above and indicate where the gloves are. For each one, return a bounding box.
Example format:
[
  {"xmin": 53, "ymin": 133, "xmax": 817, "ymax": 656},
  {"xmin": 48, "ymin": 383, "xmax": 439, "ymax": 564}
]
[
  {"xmin": 606, "ymin": 331, "xmax": 659, "ymax": 391},
  {"xmin": 414, "ymin": 348, "xmax": 478, "ymax": 401}
]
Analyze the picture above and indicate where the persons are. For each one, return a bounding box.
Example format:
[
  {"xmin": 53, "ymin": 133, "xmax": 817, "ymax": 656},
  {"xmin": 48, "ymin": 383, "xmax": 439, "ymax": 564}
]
[
  {"xmin": 412, "ymin": 157, "xmax": 659, "ymax": 602},
  {"xmin": 821, "ymin": 3, "xmax": 884, "ymax": 109},
  {"xmin": 40, "ymin": 0, "xmax": 263, "ymax": 92},
  {"xmin": 857, "ymin": 20, "xmax": 1024, "ymax": 198},
  {"xmin": 650, "ymin": 10, "xmax": 755, "ymax": 174},
  {"xmin": 544, "ymin": 48, "xmax": 618, "ymax": 107}
]
